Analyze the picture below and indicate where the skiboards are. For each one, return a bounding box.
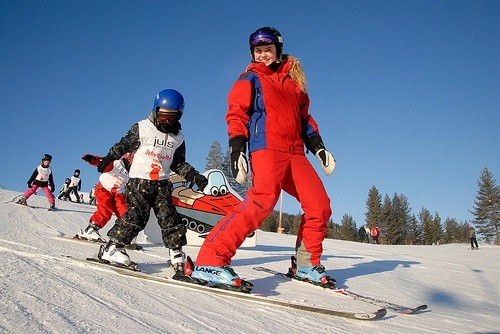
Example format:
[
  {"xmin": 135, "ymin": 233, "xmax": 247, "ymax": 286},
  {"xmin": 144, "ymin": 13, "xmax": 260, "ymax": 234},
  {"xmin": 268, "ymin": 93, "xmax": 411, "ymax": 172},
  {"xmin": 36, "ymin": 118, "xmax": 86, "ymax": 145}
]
[
  {"xmin": 251, "ymin": 264, "xmax": 431, "ymax": 315},
  {"xmin": 16, "ymin": 202, "xmax": 58, "ymax": 213},
  {"xmin": 71, "ymin": 235, "xmax": 145, "ymax": 250},
  {"xmin": 63, "ymin": 255, "xmax": 389, "ymax": 322}
]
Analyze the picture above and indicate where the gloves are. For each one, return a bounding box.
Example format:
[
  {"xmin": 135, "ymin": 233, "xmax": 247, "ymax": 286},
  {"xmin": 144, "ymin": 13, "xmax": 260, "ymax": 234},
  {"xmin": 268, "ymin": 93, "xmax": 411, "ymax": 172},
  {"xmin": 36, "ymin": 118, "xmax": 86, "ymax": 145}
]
[
  {"xmin": 60, "ymin": 191, "xmax": 62, "ymax": 193},
  {"xmin": 82, "ymin": 153, "xmax": 96, "ymax": 163},
  {"xmin": 229, "ymin": 134, "xmax": 249, "ymax": 183},
  {"xmin": 28, "ymin": 183, "xmax": 32, "ymax": 188},
  {"xmin": 311, "ymin": 141, "xmax": 336, "ymax": 176},
  {"xmin": 78, "ymin": 188, "xmax": 80, "ymax": 191},
  {"xmin": 51, "ymin": 188, "xmax": 55, "ymax": 192},
  {"xmin": 97, "ymin": 155, "xmax": 113, "ymax": 174}
]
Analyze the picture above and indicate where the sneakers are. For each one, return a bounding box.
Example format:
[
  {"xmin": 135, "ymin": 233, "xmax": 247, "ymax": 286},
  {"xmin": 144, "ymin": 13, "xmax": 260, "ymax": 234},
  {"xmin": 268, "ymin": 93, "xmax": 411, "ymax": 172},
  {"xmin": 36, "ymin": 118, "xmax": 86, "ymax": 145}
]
[
  {"xmin": 170, "ymin": 248, "xmax": 186, "ymax": 266},
  {"xmin": 296, "ymin": 265, "xmax": 337, "ymax": 286},
  {"xmin": 102, "ymin": 242, "xmax": 131, "ymax": 266},
  {"xmin": 85, "ymin": 226, "xmax": 101, "ymax": 239},
  {"xmin": 191, "ymin": 264, "xmax": 241, "ymax": 287}
]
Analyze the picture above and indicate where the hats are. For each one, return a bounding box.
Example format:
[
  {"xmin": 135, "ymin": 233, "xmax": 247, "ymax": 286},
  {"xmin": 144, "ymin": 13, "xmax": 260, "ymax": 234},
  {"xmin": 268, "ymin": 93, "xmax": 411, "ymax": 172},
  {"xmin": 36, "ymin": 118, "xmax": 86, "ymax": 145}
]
[
  {"xmin": 42, "ymin": 154, "xmax": 52, "ymax": 162},
  {"xmin": 75, "ymin": 168, "xmax": 80, "ymax": 175},
  {"xmin": 65, "ymin": 178, "xmax": 70, "ymax": 183}
]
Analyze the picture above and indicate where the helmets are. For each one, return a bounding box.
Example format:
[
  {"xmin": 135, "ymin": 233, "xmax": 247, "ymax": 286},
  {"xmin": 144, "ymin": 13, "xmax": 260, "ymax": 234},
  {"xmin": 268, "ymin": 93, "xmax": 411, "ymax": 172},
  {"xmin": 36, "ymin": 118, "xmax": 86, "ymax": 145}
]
[
  {"xmin": 249, "ymin": 26, "xmax": 284, "ymax": 63},
  {"xmin": 153, "ymin": 87, "xmax": 185, "ymax": 120}
]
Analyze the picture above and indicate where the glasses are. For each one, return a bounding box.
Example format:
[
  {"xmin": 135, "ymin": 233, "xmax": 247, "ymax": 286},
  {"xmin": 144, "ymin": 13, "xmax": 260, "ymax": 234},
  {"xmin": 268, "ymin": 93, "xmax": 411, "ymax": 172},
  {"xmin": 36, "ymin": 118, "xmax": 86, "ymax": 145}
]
[{"xmin": 156, "ymin": 112, "xmax": 178, "ymax": 123}]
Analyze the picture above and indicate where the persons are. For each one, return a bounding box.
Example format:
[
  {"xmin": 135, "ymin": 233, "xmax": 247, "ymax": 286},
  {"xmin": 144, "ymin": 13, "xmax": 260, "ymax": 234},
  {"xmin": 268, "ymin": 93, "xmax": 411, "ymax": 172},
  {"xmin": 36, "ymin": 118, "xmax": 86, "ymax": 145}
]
[
  {"xmin": 357, "ymin": 225, "xmax": 380, "ymax": 244},
  {"xmin": 193, "ymin": 26, "xmax": 337, "ymax": 288},
  {"xmin": 97, "ymin": 90, "xmax": 207, "ymax": 266},
  {"xmin": 468, "ymin": 224, "xmax": 479, "ymax": 248},
  {"xmin": 58, "ymin": 169, "xmax": 97, "ymax": 205},
  {"xmin": 78, "ymin": 152, "xmax": 135, "ymax": 244},
  {"xmin": 19, "ymin": 154, "xmax": 55, "ymax": 209}
]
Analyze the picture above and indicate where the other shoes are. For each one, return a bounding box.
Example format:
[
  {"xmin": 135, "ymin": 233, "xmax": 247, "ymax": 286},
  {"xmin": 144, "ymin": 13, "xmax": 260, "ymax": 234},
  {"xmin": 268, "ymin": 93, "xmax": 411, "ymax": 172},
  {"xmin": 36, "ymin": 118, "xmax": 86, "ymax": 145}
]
[
  {"xmin": 49, "ymin": 203, "xmax": 56, "ymax": 209},
  {"xmin": 19, "ymin": 197, "xmax": 26, "ymax": 203}
]
